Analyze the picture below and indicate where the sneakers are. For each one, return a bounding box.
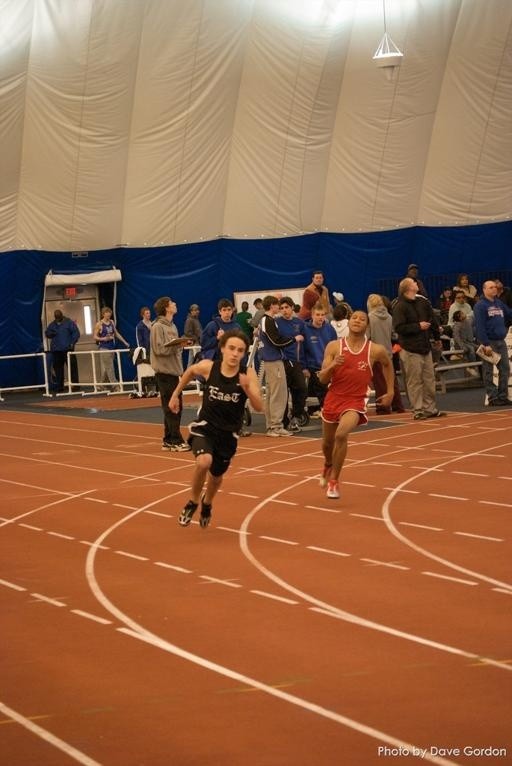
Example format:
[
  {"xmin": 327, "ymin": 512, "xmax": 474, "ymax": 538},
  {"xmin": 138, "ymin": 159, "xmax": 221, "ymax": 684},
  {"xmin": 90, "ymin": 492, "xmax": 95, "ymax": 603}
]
[
  {"xmin": 176, "ymin": 498, "xmax": 199, "ymax": 527},
  {"xmin": 236, "ymin": 428, "xmax": 253, "ymax": 437},
  {"xmin": 170, "ymin": 442, "xmax": 192, "ymax": 453},
  {"xmin": 413, "ymin": 412, "xmax": 427, "ymax": 420},
  {"xmin": 326, "ymin": 478, "xmax": 341, "ymax": 499},
  {"xmin": 265, "ymin": 414, "xmax": 303, "ymax": 437},
  {"xmin": 198, "ymin": 491, "xmax": 213, "ymax": 528},
  {"xmin": 319, "ymin": 463, "xmax": 333, "ymax": 489},
  {"xmin": 159, "ymin": 440, "xmax": 173, "ymax": 451},
  {"xmin": 486, "ymin": 396, "xmax": 512, "ymax": 406},
  {"xmin": 423, "ymin": 409, "xmax": 448, "ymax": 418}
]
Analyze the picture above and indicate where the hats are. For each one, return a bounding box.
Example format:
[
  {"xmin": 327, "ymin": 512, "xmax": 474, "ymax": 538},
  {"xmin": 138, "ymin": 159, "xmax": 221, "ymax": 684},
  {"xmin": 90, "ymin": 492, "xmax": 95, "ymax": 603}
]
[
  {"xmin": 331, "ymin": 291, "xmax": 344, "ymax": 302},
  {"xmin": 408, "ymin": 264, "xmax": 419, "ymax": 270}
]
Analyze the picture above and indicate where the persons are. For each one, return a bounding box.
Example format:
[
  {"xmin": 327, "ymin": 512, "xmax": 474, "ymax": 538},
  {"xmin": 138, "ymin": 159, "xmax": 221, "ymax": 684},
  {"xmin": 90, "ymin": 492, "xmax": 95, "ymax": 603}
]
[
  {"xmin": 45, "ymin": 310, "xmax": 80, "ymax": 392},
  {"xmin": 92, "ymin": 307, "xmax": 130, "ymax": 394},
  {"xmin": 149, "ymin": 295, "xmax": 191, "ymax": 452},
  {"xmin": 135, "ymin": 305, "xmax": 159, "ymax": 397},
  {"xmin": 167, "ymin": 329, "xmax": 265, "ymax": 529},
  {"xmin": 318, "ymin": 311, "xmax": 394, "ymax": 499}
]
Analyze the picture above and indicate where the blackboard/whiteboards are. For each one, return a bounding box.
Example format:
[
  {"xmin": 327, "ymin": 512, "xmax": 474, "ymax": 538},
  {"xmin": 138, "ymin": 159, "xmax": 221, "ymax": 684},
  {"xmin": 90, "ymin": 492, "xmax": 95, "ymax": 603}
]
[{"xmin": 234, "ymin": 288, "xmax": 305, "ymax": 318}]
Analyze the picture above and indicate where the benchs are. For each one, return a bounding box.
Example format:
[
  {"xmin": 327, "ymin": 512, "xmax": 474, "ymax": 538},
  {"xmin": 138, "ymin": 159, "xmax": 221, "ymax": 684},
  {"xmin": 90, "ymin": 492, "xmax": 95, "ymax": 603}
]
[{"xmin": 256, "ymin": 322, "xmax": 489, "ymax": 427}]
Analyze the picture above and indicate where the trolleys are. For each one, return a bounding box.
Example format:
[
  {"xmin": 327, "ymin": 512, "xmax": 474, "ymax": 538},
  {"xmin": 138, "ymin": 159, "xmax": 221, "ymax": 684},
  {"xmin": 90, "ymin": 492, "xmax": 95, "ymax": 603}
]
[{"xmin": 242, "ymin": 385, "xmax": 320, "ymax": 426}]
